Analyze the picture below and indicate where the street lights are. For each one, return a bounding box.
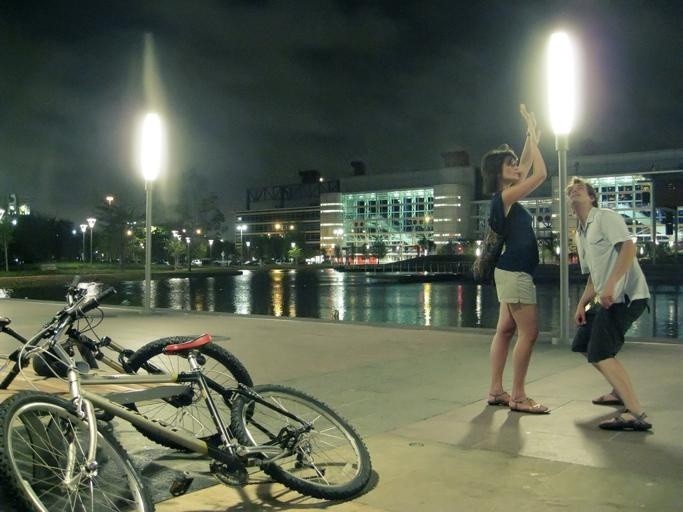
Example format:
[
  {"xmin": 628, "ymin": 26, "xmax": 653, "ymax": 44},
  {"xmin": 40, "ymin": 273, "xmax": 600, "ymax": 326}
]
[
  {"xmin": 137, "ymin": 111, "xmax": 168, "ymax": 309},
  {"xmin": 542, "ymin": 25, "xmax": 584, "ymax": 347}
]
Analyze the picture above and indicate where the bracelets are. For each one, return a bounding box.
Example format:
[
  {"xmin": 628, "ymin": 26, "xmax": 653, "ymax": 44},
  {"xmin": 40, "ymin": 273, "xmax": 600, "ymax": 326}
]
[{"xmin": 525, "ymin": 131, "xmax": 531, "ymax": 138}]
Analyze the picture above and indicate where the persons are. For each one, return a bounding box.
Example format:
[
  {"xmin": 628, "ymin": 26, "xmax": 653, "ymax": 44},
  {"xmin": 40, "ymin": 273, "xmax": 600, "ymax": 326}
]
[
  {"xmin": 476, "ymin": 102, "xmax": 550, "ymax": 417},
  {"xmin": 563, "ymin": 176, "xmax": 654, "ymax": 432}
]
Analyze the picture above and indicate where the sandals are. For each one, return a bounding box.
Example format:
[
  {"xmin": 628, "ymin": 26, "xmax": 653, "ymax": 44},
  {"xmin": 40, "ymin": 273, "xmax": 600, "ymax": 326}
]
[
  {"xmin": 599, "ymin": 409, "xmax": 652, "ymax": 430},
  {"xmin": 592, "ymin": 392, "xmax": 623, "ymax": 405},
  {"xmin": 487, "ymin": 392, "xmax": 510, "ymax": 405},
  {"xmin": 509, "ymin": 397, "xmax": 550, "ymax": 414}
]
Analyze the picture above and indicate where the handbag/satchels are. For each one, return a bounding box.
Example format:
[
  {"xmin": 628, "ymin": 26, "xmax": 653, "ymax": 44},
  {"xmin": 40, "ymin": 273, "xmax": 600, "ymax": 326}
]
[{"xmin": 470, "ymin": 237, "xmax": 502, "ymax": 288}]
